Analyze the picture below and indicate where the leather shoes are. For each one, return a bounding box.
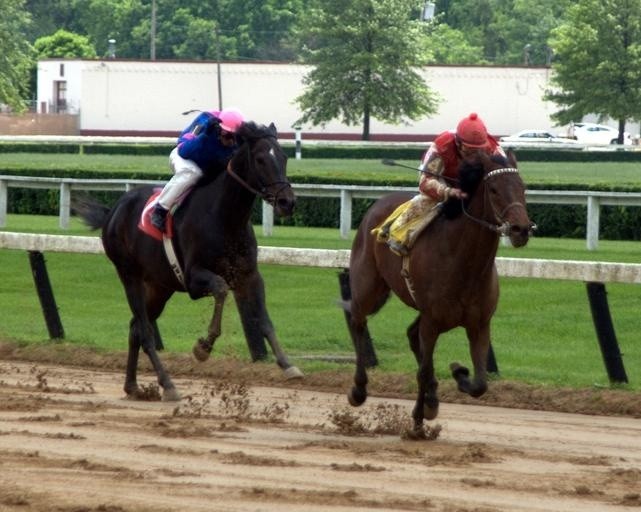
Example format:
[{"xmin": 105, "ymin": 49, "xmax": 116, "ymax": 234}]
[
  {"xmin": 386, "ymin": 238, "xmax": 409, "ymax": 257},
  {"xmin": 150, "ymin": 202, "xmax": 169, "ymax": 234}
]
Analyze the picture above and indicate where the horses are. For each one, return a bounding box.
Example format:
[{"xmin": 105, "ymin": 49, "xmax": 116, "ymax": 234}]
[
  {"xmin": 350, "ymin": 146, "xmax": 533, "ymax": 439},
  {"xmin": 68, "ymin": 120, "xmax": 306, "ymax": 403}
]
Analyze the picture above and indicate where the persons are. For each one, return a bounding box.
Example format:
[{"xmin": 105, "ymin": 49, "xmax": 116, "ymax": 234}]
[
  {"xmin": 151, "ymin": 109, "xmax": 244, "ymax": 231},
  {"xmin": 386, "ymin": 112, "xmax": 506, "ymax": 256}
]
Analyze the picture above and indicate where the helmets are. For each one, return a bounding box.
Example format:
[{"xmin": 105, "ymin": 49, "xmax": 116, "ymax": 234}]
[
  {"xmin": 217, "ymin": 110, "xmax": 242, "ymax": 134},
  {"xmin": 456, "ymin": 113, "xmax": 490, "ymax": 149}
]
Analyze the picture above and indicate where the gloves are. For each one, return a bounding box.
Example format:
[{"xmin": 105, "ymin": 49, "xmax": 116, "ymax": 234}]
[{"xmin": 205, "ymin": 117, "xmax": 222, "ymax": 136}]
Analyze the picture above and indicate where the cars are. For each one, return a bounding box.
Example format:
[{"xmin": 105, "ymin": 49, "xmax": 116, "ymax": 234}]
[
  {"xmin": 501, "ymin": 130, "xmax": 585, "ymax": 150},
  {"xmin": 575, "ymin": 123, "xmax": 632, "ymax": 146}
]
